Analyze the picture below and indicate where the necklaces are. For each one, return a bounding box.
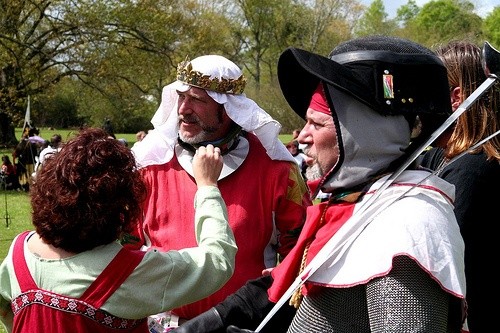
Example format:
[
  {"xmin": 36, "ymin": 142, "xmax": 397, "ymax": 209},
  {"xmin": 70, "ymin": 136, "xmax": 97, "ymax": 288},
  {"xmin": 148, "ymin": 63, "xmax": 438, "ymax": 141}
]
[{"xmin": 288, "ymin": 172, "xmax": 393, "ymax": 311}]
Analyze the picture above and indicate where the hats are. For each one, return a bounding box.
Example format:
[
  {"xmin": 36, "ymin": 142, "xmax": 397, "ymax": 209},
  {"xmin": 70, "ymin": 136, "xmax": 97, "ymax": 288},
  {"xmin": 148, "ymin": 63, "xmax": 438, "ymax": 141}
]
[{"xmin": 277, "ymin": 36, "xmax": 453, "ymax": 140}]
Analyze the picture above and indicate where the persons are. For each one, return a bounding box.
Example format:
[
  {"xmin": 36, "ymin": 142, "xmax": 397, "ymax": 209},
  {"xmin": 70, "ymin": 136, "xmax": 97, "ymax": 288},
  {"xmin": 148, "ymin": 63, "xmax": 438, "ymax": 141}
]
[
  {"xmin": 0, "ymin": 127, "xmax": 66, "ymax": 192},
  {"xmin": 135, "ymin": 36, "xmax": 500, "ymax": 333},
  {"xmin": 0, "ymin": 126, "xmax": 238, "ymax": 333}
]
